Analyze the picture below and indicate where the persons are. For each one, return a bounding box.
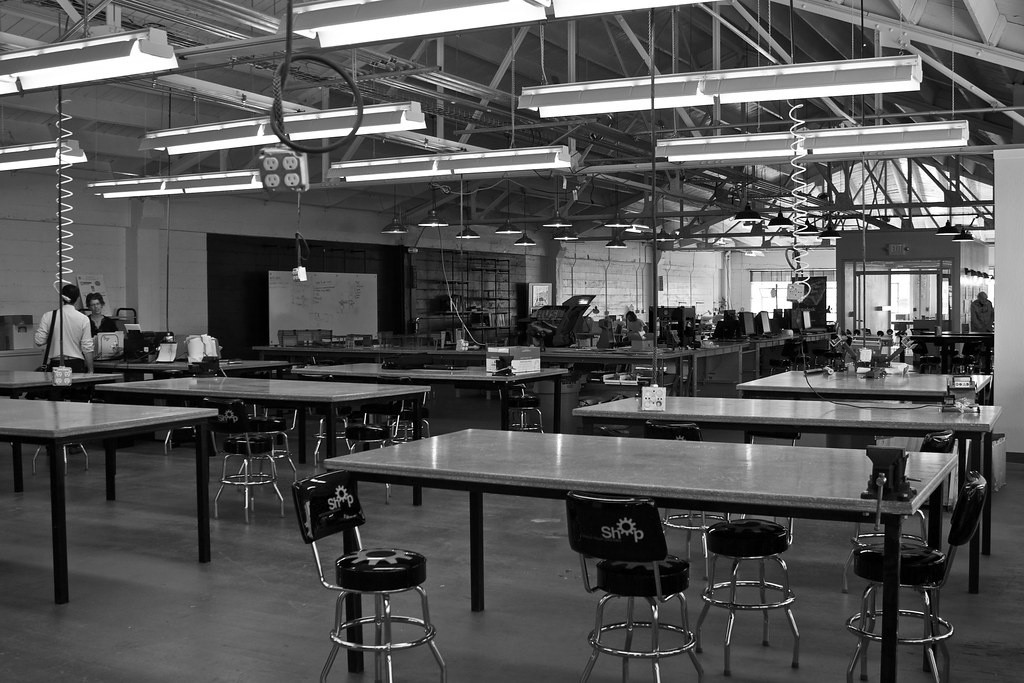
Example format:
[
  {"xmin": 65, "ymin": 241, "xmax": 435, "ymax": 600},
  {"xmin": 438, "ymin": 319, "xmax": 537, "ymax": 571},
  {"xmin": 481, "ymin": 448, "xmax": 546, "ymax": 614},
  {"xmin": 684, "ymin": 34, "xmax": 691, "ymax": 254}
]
[
  {"xmin": 626, "ymin": 311, "xmax": 649, "ymax": 333},
  {"xmin": 971, "ymin": 292, "xmax": 994, "ymax": 332},
  {"xmin": 86, "ymin": 293, "xmax": 119, "ymax": 338},
  {"xmin": 33, "ymin": 284, "xmax": 95, "ymax": 374}
]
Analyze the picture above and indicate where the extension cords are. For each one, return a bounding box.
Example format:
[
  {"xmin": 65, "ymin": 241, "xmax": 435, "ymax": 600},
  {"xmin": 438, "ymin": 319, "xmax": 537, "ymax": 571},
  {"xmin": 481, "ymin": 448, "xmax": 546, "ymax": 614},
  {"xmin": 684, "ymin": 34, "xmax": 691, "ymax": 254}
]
[{"xmin": 256, "ymin": 147, "xmax": 310, "ymax": 192}]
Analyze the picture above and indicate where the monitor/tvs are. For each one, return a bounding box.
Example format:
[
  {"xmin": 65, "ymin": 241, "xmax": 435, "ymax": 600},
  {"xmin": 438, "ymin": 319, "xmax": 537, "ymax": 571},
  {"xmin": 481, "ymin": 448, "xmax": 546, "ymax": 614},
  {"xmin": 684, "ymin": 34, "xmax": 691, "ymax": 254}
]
[
  {"xmin": 760, "ymin": 312, "xmax": 770, "ymax": 334},
  {"xmin": 802, "ymin": 311, "xmax": 812, "ymax": 329},
  {"xmin": 738, "ymin": 312, "xmax": 754, "ymax": 334}
]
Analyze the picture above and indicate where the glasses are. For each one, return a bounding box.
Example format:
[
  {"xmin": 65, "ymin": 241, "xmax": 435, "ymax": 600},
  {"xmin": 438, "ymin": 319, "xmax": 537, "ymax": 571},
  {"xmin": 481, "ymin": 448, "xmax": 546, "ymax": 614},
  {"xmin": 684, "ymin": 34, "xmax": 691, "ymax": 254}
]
[{"xmin": 90, "ymin": 302, "xmax": 101, "ymax": 308}]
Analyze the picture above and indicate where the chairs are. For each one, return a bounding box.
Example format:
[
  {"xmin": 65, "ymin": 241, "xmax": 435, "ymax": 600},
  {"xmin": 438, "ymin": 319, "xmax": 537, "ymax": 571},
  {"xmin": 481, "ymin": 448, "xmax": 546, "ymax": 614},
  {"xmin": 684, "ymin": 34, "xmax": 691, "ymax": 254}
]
[
  {"xmin": 499, "ymin": 384, "xmax": 545, "ymax": 433},
  {"xmin": 695, "ymin": 513, "xmax": 801, "ymax": 676},
  {"xmin": 291, "ymin": 469, "xmax": 448, "ymax": 683},
  {"xmin": 843, "ymin": 430, "xmax": 956, "ymax": 593},
  {"xmin": 247, "ymin": 405, "xmax": 297, "ymax": 459},
  {"xmin": 644, "ymin": 419, "xmax": 729, "ymax": 560},
  {"xmin": 346, "ymin": 400, "xmax": 404, "ymax": 506},
  {"xmin": 165, "ymin": 426, "xmax": 218, "ymax": 456},
  {"xmin": 845, "ymin": 472, "xmax": 988, "ymax": 683},
  {"xmin": 204, "ymin": 397, "xmax": 284, "ymax": 523},
  {"xmin": 32, "ymin": 443, "xmax": 89, "ymax": 476},
  {"xmin": 309, "ymin": 406, "xmax": 351, "ymax": 467},
  {"xmin": 566, "ymin": 489, "xmax": 704, "ymax": 683}
]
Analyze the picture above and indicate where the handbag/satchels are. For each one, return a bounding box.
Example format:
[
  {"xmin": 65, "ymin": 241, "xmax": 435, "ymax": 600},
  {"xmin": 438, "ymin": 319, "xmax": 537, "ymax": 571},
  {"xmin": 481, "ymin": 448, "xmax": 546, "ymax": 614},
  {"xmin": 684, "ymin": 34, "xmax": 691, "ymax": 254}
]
[{"xmin": 25, "ymin": 365, "xmax": 48, "ymax": 401}]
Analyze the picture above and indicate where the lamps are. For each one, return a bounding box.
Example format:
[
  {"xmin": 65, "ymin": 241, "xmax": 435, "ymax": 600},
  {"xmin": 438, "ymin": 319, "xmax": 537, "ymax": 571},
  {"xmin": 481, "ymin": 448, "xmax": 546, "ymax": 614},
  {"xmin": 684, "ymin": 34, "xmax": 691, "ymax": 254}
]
[
  {"xmin": 0, "ymin": 138, "xmax": 88, "ymax": 173},
  {"xmin": 456, "ymin": 181, "xmax": 481, "ymax": 239},
  {"xmin": 768, "ymin": 164, "xmax": 794, "ymax": 226},
  {"xmin": 516, "ymin": 0, "xmax": 924, "ymax": 117},
  {"xmin": 495, "ymin": 178, "xmax": 522, "ymax": 234},
  {"xmin": 417, "ymin": 183, "xmax": 449, "ymax": 227},
  {"xmin": 553, "ymin": 228, "xmax": 578, "ymax": 240},
  {"xmin": 604, "ymin": 173, "xmax": 631, "ymax": 227},
  {"xmin": 934, "ymin": 155, "xmax": 961, "ymax": 235},
  {"xmin": 818, "ymin": 162, "xmax": 842, "ymax": 239},
  {"xmin": 797, "ymin": 163, "xmax": 819, "ymax": 233},
  {"xmin": 542, "ymin": 175, "xmax": 572, "ymax": 227},
  {"xmin": 0, "ymin": 0, "xmax": 179, "ymax": 95},
  {"xmin": 137, "ymin": 100, "xmax": 429, "ymax": 155},
  {"xmin": 290, "ymin": 0, "xmax": 733, "ymax": 47},
  {"xmin": 326, "ymin": 145, "xmax": 571, "ymax": 184},
  {"xmin": 605, "ymin": 228, "xmax": 628, "ymax": 249},
  {"xmin": 380, "ymin": 185, "xmax": 408, "ymax": 233},
  {"xmin": 656, "ymin": 197, "xmax": 675, "ymax": 241},
  {"xmin": 87, "ymin": 168, "xmax": 264, "ymax": 199},
  {"xmin": 514, "ymin": 192, "xmax": 536, "ymax": 246},
  {"xmin": 734, "ymin": 165, "xmax": 763, "ymax": 221},
  {"xmin": 654, "ymin": 0, "xmax": 969, "ymax": 163},
  {"xmin": 952, "ymin": 227, "xmax": 974, "ymax": 242}
]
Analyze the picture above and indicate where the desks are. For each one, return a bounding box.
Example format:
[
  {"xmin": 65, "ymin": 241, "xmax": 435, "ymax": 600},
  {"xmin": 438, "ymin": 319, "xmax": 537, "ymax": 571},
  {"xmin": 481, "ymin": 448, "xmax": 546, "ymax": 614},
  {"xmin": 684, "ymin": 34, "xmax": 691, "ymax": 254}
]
[
  {"xmin": 736, "ymin": 370, "xmax": 993, "ymax": 495},
  {"xmin": 692, "ymin": 340, "xmax": 750, "ymax": 396},
  {"xmin": 799, "ymin": 328, "xmax": 831, "ymax": 368},
  {"xmin": 750, "ymin": 336, "xmax": 798, "ymax": 379},
  {"xmin": 0, "ymin": 333, "xmax": 1003, "ymax": 683}
]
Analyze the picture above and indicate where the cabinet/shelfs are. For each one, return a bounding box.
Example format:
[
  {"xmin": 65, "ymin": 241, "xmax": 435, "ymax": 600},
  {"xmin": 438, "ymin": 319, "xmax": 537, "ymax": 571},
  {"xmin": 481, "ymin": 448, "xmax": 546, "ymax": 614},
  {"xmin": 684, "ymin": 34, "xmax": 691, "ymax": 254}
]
[{"xmin": 433, "ymin": 251, "xmax": 511, "ymax": 346}]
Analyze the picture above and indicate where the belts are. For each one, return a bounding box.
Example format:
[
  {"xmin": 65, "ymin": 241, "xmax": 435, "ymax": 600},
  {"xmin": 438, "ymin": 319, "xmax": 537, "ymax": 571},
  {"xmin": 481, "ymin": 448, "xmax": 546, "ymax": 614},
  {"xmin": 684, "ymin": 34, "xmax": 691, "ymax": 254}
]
[{"xmin": 51, "ymin": 355, "xmax": 77, "ymax": 360}]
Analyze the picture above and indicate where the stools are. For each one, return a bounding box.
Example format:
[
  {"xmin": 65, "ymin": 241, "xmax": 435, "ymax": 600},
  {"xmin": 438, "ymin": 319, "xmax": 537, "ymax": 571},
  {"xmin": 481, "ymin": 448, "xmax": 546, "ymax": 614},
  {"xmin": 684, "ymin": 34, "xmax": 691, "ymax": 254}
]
[
  {"xmin": 951, "ymin": 354, "xmax": 975, "ymax": 374},
  {"xmin": 918, "ymin": 355, "xmax": 941, "ymax": 374}
]
